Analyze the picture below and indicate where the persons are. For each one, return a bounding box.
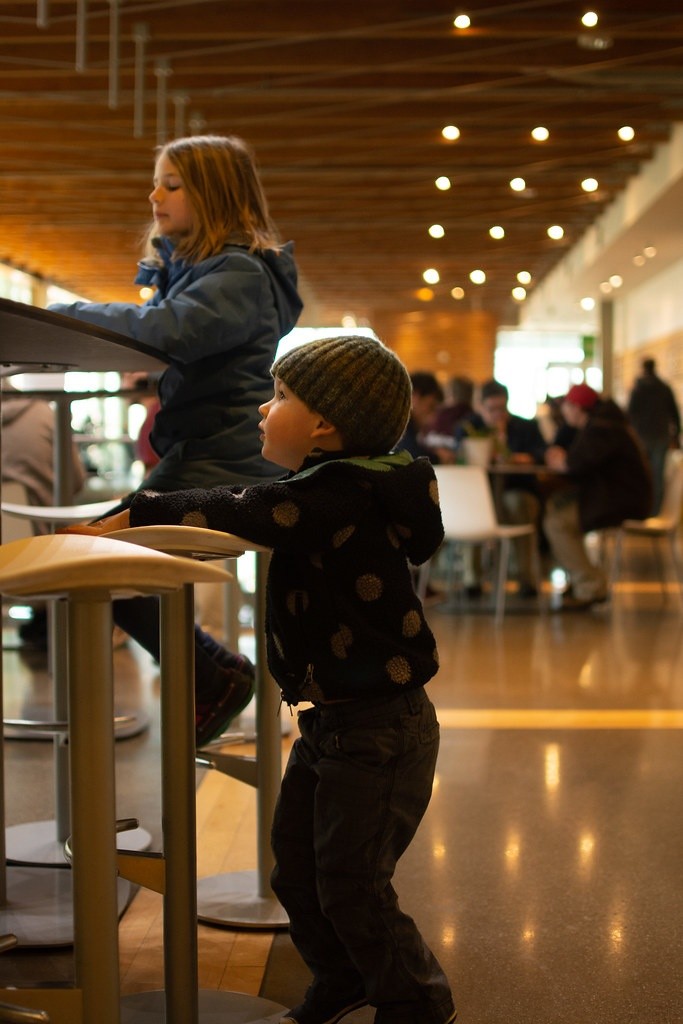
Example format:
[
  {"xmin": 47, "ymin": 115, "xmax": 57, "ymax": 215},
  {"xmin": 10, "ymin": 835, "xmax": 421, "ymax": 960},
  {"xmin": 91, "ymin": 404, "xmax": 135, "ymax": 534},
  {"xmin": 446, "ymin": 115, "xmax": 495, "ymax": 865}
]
[
  {"xmin": 402, "ymin": 359, "xmax": 682, "ymax": 611},
  {"xmin": 46, "ymin": 136, "xmax": 304, "ymax": 750},
  {"xmin": 0, "ymin": 365, "xmax": 163, "ymax": 720},
  {"xmin": 58, "ymin": 336, "xmax": 457, "ymax": 1024}
]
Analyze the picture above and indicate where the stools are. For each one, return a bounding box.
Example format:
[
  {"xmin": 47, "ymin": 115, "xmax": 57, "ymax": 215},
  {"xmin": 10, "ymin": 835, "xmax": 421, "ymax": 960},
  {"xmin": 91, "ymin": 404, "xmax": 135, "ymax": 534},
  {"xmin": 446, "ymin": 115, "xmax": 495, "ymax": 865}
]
[
  {"xmin": 98, "ymin": 524, "xmax": 292, "ymax": 1024},
  {"xmin": 0, "ymin": 499, "xmax": 150, "ymax": 866},
  {"xmin": 196, "ymin": 535, "xmax": 293, "ymax": 936},
  {"xmin": 0, "ymin": 534, "xmax": 233, "ymax": 1024}
]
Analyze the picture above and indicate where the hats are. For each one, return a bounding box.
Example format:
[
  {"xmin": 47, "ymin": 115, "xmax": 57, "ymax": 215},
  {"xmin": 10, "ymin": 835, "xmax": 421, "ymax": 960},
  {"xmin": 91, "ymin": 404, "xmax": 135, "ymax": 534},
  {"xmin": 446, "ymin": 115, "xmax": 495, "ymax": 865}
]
[
  {"xmin": 565, "ymin": 384, "xmax": 598, "ymax": 410},
  {"xmin": 271, "ymin": 336, "xmax": 413, "ymax": 457}
]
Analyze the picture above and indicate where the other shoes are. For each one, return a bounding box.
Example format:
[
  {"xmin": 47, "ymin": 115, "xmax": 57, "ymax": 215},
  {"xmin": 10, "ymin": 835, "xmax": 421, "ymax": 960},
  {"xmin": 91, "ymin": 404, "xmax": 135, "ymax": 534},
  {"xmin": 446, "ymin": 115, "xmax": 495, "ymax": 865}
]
[
  {"xmin": 415, "ymin": 572, "xmax": 436, "ymax": 597},
  {"xmin": 515, "ymin": 583, "xmax": 540, "ymax": 599},
  {"xmin": 193, "ymin": 656, "xmax": 257, "ymax": 753},
  {"xmin": 562, "ymin": 585, "xmax": 578, "ymax": 598},
  {"xmin": 558, "ymin": 596, "xmax": 606, "ymax": 615},
  {"xmin": 465, "ymin": 585, "xmax": 482, "ymax": 600},
  {"xmin": 277, "ymin": 978, "xmax": 371, "ymax": 1024},
  {"xmin": 19, "ymin": 611, "xmax": 48, "ymax": 642}
]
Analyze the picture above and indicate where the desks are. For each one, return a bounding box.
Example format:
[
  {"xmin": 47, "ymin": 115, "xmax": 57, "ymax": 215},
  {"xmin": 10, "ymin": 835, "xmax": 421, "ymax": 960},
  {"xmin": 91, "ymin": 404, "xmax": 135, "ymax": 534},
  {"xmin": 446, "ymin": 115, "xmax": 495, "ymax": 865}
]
[
  {"xmin": 0, "ymin": 296, "xmax": 175, "ymax": 989},
  {"xmin": 430, "ymin": 464, "xmax": 551, "ymax": 616},
  {"xmin": 0, "ymin": 371, "xmax": 138, "ymax": 743}
]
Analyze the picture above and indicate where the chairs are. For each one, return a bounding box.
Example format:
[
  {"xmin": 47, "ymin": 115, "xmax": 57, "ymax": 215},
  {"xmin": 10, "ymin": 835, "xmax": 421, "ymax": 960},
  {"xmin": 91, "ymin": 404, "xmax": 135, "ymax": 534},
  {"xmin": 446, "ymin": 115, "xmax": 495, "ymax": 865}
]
[
  {"xmin": 598, "ymin": 450, "xmax": 683, "ymax": 609},
  {"xmin": 432, "ymin": 466, "xmax": 547, "ymax": 626}
]
